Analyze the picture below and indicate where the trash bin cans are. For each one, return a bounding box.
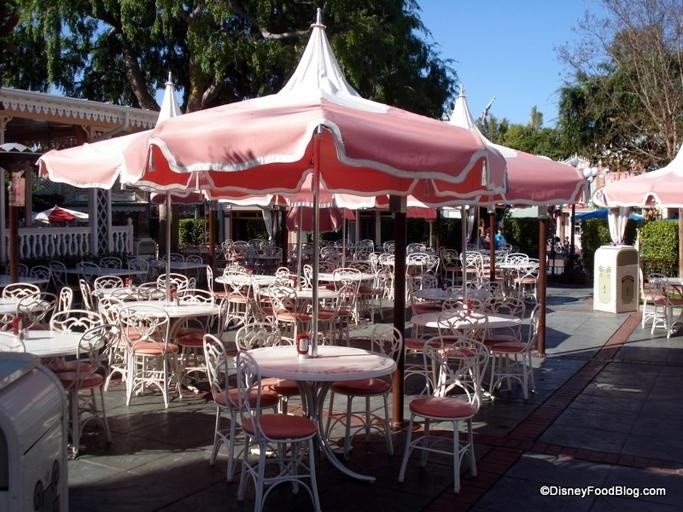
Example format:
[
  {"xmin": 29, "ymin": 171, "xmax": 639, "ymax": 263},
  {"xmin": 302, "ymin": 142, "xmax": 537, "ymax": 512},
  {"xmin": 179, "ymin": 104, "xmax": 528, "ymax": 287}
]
[
  {"xmin": 593, "ymin": 245, "xmax": 638, "ymax": 313},
  {"xmin": 0, "ymin": 352, "xmax": 70, "ymax": 512}
]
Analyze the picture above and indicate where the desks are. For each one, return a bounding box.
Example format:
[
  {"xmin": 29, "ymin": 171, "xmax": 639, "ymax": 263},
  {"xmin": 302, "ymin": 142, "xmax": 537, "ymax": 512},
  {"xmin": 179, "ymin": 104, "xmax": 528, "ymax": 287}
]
[{"xmin": 650, "ymin": 278, "xmax": 683, "ymax": 334}]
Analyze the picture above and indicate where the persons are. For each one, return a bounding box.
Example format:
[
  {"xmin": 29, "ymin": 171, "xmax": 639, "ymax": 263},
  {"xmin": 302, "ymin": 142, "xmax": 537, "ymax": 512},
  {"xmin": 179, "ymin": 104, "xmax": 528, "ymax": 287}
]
[{"xmin": 32, "ymin": 7, "xmax": 683, "ymax": 471}]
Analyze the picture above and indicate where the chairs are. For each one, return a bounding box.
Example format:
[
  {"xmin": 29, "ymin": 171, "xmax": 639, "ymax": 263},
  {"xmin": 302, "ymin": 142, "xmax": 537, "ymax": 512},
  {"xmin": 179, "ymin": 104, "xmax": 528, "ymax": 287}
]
[
  {"xmin": 639, "ymin": 267, "xmax": 668, "ymax": 330},
  {"xmin": 648, "ymin": 272, "xmax": 683, "ymax": 339},
  {"xmin": 0, "ymin": 240, "xmax": 543, "ymax": 512}
]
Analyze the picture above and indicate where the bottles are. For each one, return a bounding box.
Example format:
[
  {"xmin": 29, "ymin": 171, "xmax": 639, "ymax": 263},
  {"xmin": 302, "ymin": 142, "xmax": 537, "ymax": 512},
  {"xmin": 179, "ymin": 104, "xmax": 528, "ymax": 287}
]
[
  {"xmin": 125, "ymin": 278, "xmax": 132, "ymax": 288},
  {"xmin": 296, "ymin": 333, "xmax": 309, "ymax": 359},
  {"xmin": 155, "ymin": 244, "xmax": 159, "ymax": 261},
  {"xmin": 291, "ymin": 276, "xmax": 297, "ymax": 288},
  {"xmin": 463, "ymin": 302, "xmax": 471, "ymax": 316},
  {"xmin": 13, "ymin": 317, "xmax": 22, "ymax": 335}
]
